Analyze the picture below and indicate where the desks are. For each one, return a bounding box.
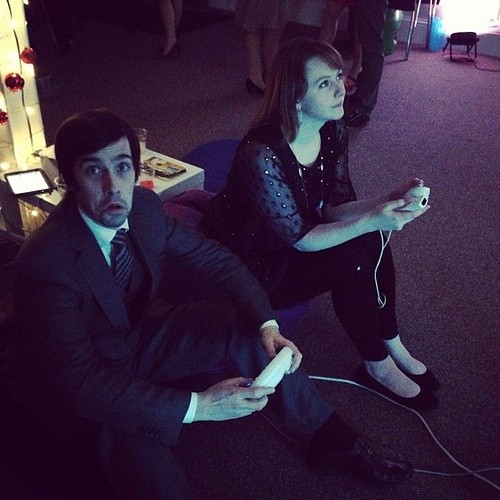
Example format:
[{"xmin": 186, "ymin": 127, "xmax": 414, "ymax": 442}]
[{"xmin": 0, "ymin": 143, "xmax": 205, "ymax": 237}]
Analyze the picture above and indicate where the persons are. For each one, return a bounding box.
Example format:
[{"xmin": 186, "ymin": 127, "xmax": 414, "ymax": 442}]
[
  {"xmin": 234, "ymin": 0, "xmax": 415, "ymax": 127},
  {"xmin": 13, "ymin": 110, "xmax": 413, "ymax": 500},
  {"xmin": 152, "ymin": 0, "xmax": 184, "ymax": 60},
  {"xmin": 225, "ymin": 40, "xmax": 441, "ymax": 413}
]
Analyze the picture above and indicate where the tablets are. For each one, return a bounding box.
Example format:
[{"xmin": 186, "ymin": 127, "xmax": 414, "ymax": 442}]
[{"xmin": 4, "ymin": 168, "xmax": 54, "ymax": 198}]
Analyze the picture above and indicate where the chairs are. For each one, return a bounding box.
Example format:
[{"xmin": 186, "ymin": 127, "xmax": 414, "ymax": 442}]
[
  {"xmin": 443, "ymin": 32, "xmax": 480, "ymax": 58},
  {"xmin": 387, "ymin": 0, "xmax": 440, "ymax": 61}
]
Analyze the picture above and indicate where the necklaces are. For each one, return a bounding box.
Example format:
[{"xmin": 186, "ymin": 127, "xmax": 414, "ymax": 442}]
[{"xmin": 278, "ymin": 124, "xmax": 325, "ymax": 217}]
